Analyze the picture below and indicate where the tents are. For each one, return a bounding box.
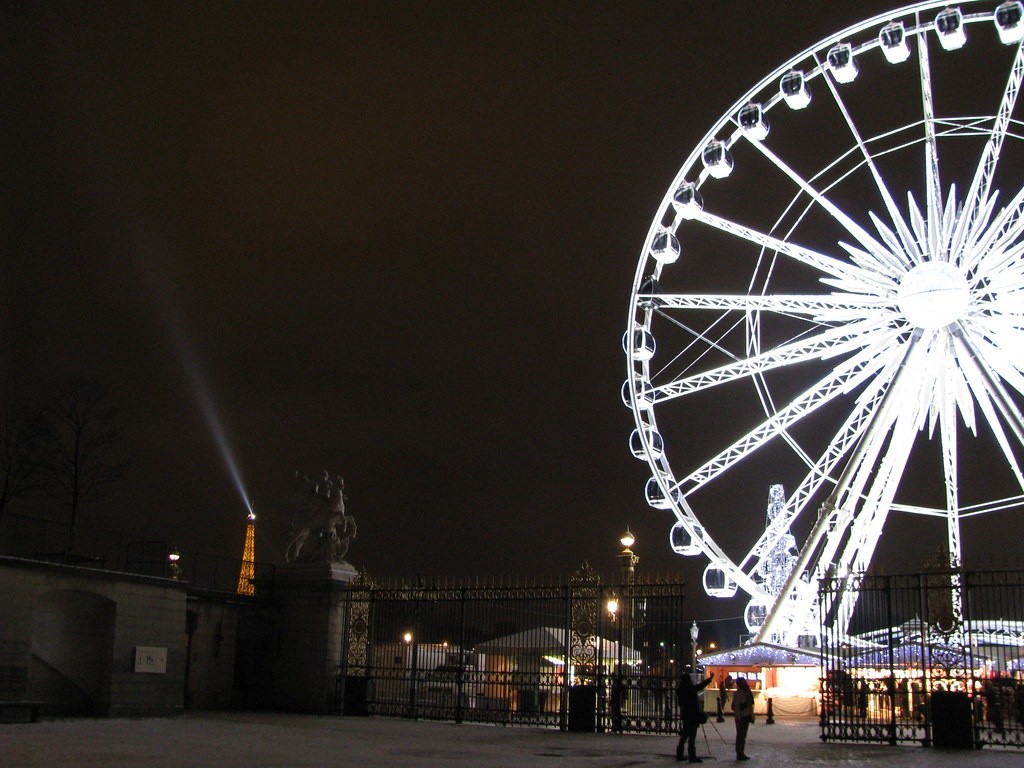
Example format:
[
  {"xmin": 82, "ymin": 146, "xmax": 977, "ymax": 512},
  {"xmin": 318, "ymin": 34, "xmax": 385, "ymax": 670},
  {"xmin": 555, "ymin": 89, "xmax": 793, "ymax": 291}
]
[
  {"xmin": 697, "ymin": 641, "xmax": 1023, "ymax": 722},
  {"xmin": 474, "ymin": 626, "xmax": 641, "ymax": 717}
]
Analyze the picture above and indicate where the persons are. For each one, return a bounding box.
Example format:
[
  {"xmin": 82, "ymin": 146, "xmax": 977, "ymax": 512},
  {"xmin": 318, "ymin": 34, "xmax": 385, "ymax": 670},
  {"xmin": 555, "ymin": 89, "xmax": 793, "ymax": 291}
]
[
  {"xmin": 675, "ymin": 671, "xmax": 716, "ymax": 764},
  {"xmin": 729, "ymin": 677, "xmax": 757, "ymax": 761},
  {"xmin": 725, "ymin": 675, "xmax": 733, "ymax": 689},
  {"xmin": 292, "ymin": 464, "xmax": 349, "ymax": 533},
  {"xmin": 719, "ymin": 682, "xmax": 729, "ymax": 711},
  {"xmin": 582, "ymin": 661, "xmax": 673, "ymax": 734},
  {"xmin": 822, "ymin": 657, "xmax": 1024, "ymax": 739}
]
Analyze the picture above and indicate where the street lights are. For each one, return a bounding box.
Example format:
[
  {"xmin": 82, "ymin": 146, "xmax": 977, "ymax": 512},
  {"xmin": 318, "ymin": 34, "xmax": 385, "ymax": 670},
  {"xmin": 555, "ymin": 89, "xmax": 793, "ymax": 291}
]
[
  {"xmin": 643, "ymin": 640, "xmax": 648, "ymax": 676},
  {"xmin": 169, "ymin": 545, "xmax": 180, "ymax": 579},
  {"xmin": 616, "ymin": 524, "xmax": 640, "ymax": 660},
  {"xmin": 690, "ymin": 620, "xmax": 699, "ymax": 672},
  {"xmin": 606, "ymin": 593, "xmax": 623, "ymax": 675}
]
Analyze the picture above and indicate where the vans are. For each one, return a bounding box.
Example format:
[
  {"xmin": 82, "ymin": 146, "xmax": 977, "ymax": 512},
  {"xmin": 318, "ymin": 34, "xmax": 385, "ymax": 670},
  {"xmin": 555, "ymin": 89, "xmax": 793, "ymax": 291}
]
[{"xmin": 431, "ymin": 664, "xmax": 475, "ymax": 682}]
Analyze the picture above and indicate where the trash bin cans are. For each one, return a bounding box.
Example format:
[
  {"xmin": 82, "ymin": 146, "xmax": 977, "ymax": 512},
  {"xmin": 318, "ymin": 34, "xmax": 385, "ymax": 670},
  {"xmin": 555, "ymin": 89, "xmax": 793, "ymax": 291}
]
[
  {"xmin": 931, "ymin": 691, "xmax": 973, "ymax": 751},
  {"xmin": 568, "ymin": 685, "xmax": 596, "ymax": 733}
]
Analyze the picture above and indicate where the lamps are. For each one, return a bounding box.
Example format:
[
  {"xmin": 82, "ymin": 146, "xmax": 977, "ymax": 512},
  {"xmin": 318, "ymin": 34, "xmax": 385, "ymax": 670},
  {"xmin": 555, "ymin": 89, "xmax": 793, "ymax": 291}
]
[{"xmin": 168, "ymin": 545, "xmax": 181, "ymax": 581}]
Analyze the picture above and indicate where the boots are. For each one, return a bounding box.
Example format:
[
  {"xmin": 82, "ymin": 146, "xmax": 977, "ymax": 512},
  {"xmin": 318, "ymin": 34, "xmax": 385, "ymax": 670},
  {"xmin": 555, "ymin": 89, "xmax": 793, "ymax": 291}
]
[
  {"xmin": 688, "ymin": 746, "xmax": 703, "ymax": 763},
  {"xmin": 676, "ymin": 746, "xmax": 687, "ymax": 761}
]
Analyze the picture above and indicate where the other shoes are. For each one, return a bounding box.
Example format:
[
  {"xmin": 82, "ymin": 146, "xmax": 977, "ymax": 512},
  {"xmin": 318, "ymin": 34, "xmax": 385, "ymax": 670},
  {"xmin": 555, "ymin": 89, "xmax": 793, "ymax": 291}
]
[
  {"xmin": 745, "ymin": 755, "xmax": 750, "ymax": 759},
  {"xmin": 737, "ymin": 755, "xmax": 747, "ymax": 760}
]
